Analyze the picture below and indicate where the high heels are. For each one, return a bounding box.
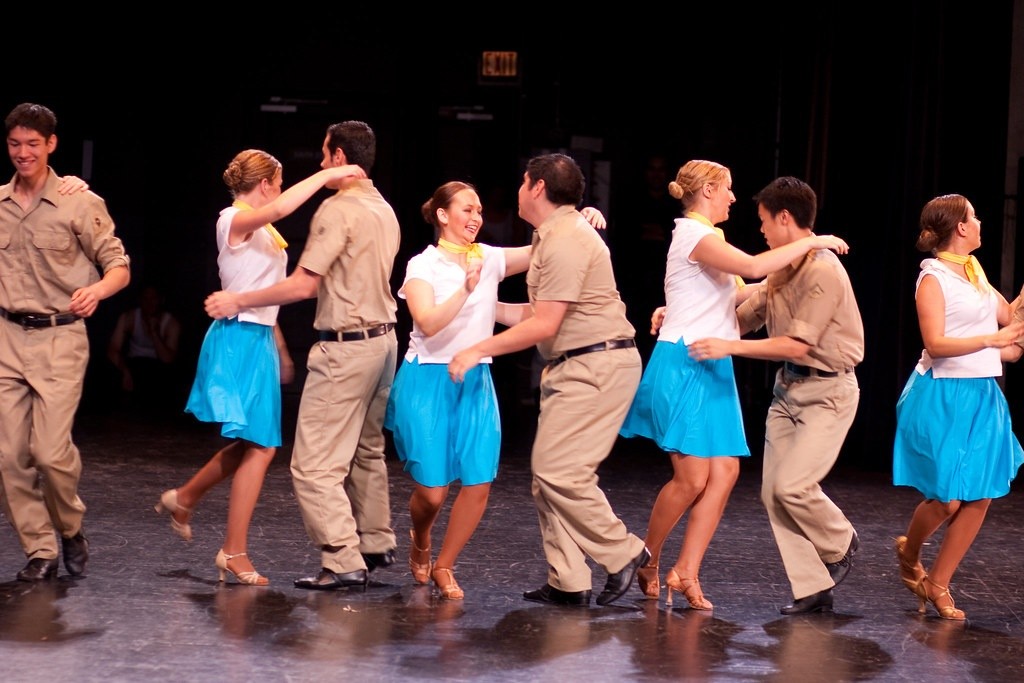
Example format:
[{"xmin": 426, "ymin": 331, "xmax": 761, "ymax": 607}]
[
  {"xmin": 895, "ymin": 534, "xmax": 926, "ymax": 592},
  {"xmin": 916, "ymin": 573, "xmax": 966, "ymax": 621},
  {"xmin": 407, "ymin": 528, "xmax": 433, "ymax": 585},
  {"xmin": 429, "ymin": 561, "xmax": 465, "ymax": 600},
  {"xmin": 215, "ymin": 547, "xmax": 269, "ymax": 586},
  {"xmin": 664, "ymin": 566, "xmax": 714, "ymax": 609},
  {"xmin": 640, "ymin": 543, "xmax": 661, "ymax": 597},
  {"xmin": 154, "ymin": 490, "xmax": 191, "ymax": 542}
]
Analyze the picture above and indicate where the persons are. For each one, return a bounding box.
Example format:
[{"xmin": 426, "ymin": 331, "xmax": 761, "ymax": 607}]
[
  {"xmin": 449, "ymin": 154, "xmax": 652, "ymax": 605},
  {"xmin": 892, "ymin": 195, "xmax": 1023, "ymax": 621},
  {"xmin": 382, "ymin": 181, "xmax": 607, "ymax": 600},
  {"xmin": 106, "ymin": 284, "xmax": 181, "ymax": 394},
  {"xmin": 619, "ymin": 159, "xmax": 850, "ymax": 612},
  {"xmin": 204, "ymin": 121, "xmax": 401, "ymax": 589},
  {"xmin": 651, "ymin": 176, "xmax": 864, "ymax": 614},
  {"xmin": 0, "ymin": 102, "xmax": 131, "ymax": 580},
  {"xmin": 154, "ymin": 148, "xmax": 367, "ymax": 585},
  {"xmin": 999, "ymin": 340, "xmax": 1023, "ymax": 363}
]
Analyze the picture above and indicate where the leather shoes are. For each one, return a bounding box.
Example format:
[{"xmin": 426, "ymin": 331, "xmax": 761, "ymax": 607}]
[
  {"xmin": 828, "ymin": 530, "xmax": 859, "ymax": 585},
  {"xmin": 522, "ymin": 579, "xmax": 592, "ymax": 607},
  {"xmin": 780, "ymin": 585, "xmax": 833, "ymax": 615},
  {"xmin": 293, "ymin": 565, "xmax": 369, "ymax": 587},
  {"xmin": 17, "ymin": 555, "xmax": 59, "ymax": 581},
  {"xmin": 61, "ymin": 530, "xmax": 89, "ymax": 576},
  {"xmin": 599, "ymin": 546, "xmax": 652, "ymax": 607},
  {"xmin": 365, "ymin": 550, "xmax": 396, "ymax": 565}
]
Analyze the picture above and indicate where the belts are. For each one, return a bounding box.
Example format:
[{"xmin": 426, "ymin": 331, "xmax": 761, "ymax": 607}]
[
  {"xmin": 318, "ymin": 323, "xmax": 394, "ymax": 342},
  {"xmin": 784, "ymin": 359, "xmax": 843, "ymax": 377},
  {"xmin": 0, "ymin": 306, "xmax": 82, "ymax": 330},
  {"xmin": 555, "ymin": 339, "xmax": 636, "ymax": 367}
]
